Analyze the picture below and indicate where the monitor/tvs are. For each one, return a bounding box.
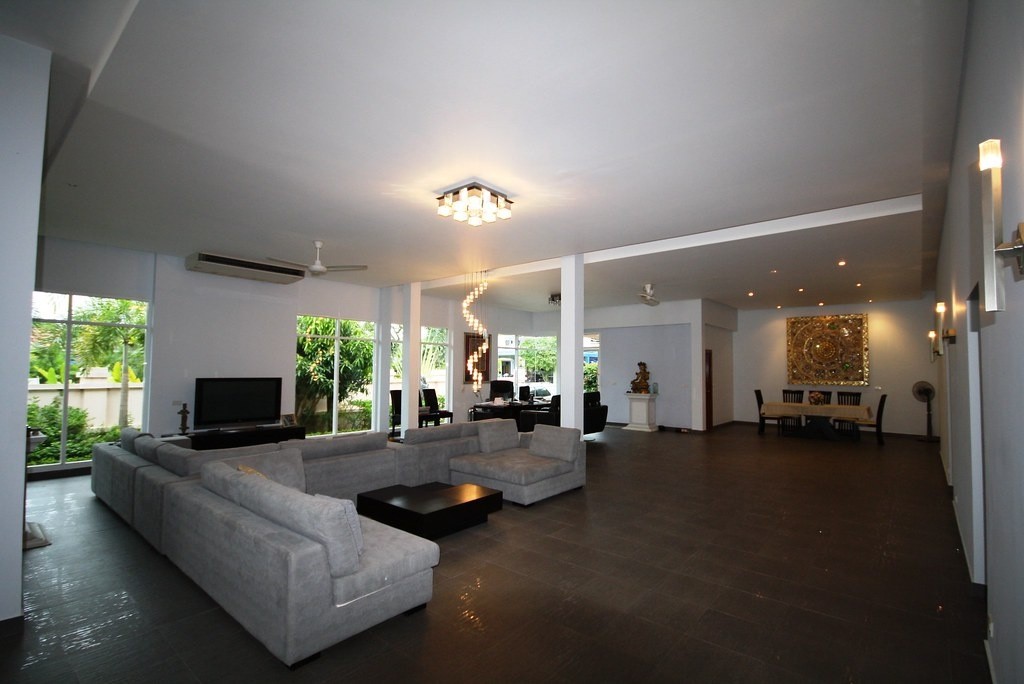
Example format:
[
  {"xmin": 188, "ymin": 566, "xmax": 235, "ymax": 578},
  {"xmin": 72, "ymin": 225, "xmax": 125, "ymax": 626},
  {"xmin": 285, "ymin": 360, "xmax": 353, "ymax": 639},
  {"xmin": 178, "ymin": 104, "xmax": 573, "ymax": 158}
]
[{"xmin": 194, "ymin": 378, "xmax": 282, "ymax": 432}]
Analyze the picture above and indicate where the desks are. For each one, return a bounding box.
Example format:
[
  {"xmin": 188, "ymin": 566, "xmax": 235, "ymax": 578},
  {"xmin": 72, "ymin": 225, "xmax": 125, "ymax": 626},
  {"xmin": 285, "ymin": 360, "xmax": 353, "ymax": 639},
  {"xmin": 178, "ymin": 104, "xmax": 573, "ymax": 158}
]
[
  {"xmin": 160, "ymin": 426, "xmax": 309, "ymax": 449},
  {"xmin": 470, "ymin": 400, "xmax": 550, "ymax": 421},
  {"xmin": 761, "ymin": 402, "xmax": 874, "ymax": 442}
]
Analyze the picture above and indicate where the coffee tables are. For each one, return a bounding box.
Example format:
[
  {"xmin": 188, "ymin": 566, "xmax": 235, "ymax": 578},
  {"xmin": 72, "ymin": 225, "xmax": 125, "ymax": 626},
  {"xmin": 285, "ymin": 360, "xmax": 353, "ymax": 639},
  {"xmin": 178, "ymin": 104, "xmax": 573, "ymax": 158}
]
[{"xmin": 358, "ymin": 483, "xmax": 503, "ymax": 548}]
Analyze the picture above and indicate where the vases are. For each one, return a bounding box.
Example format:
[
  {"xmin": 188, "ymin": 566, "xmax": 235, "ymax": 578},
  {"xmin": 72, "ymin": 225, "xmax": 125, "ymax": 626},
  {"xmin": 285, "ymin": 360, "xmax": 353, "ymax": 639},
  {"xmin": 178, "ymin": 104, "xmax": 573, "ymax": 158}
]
[{"xmin": 812, "ymin": 400, "xmax": 821, "ymax": 407}]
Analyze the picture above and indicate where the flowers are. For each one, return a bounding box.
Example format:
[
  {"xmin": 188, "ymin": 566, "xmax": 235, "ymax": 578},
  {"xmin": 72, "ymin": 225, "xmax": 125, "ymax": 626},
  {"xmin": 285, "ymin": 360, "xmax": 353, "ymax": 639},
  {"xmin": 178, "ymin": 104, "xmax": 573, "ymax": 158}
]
[{"xmin": 807, "ymin": 391, "xmax": 823, "ymax": 401}]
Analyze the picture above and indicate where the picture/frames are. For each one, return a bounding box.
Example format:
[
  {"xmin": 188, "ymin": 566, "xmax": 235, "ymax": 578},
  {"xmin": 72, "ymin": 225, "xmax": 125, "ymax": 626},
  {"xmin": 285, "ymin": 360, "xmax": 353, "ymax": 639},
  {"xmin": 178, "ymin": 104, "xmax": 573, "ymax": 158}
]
[
  {"xmin": 785, "ymin": 312, "xmax": 869, "ymax": 386},
  {"xmin": 464, "ymin": 333, "xmax": 493, "ymax": 383},
  {"xmin": 281, "ymin": 413, "xmax": 295, "ymax": 426}
]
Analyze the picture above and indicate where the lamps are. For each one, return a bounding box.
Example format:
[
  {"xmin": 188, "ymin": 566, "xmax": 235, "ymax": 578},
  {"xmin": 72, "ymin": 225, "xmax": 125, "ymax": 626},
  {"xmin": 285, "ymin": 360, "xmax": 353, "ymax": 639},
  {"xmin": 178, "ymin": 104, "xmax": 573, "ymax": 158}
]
[
  {"xmin": 979, "ymin": 138, "xmax": 1024, "ymax": 312},
  {"xmin": 929, "ymin": 330, "xmax": 942, "ymax": 364},
  {"xmin": 463, "ymin": 269, "xmax": 490, "ymax": 394},
  {"xmin": 934, "ymin": 300, "xmax": 956, "ymax": 355},
  {"xmin": 549, "ymin": 294, "xmax": 561, "ymax": 307},
  {"xmin": 435, "ymin": 182, "xmax": 514, "ymax": 227}
]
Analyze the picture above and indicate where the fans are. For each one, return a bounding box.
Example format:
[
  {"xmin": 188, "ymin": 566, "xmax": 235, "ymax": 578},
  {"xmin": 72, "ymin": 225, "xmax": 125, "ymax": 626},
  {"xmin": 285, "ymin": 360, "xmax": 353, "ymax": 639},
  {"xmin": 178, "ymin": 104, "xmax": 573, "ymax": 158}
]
[
  {"xmin": 635, "ymin": 283, "xmax": 659, "ymax": 306},
  {"xmin": 912, "ymin": 381, "xmax": 938, "ymax": 442},
  {"xmin": 267, "ymin": 239, "xmax": 368, "ymax": 276}
]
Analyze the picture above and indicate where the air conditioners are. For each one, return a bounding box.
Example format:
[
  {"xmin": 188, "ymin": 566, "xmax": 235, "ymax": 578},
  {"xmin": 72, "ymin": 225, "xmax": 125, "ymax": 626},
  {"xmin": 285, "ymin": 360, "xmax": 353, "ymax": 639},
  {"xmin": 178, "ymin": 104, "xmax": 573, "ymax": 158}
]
[{"xmin": 186, "ymin": 251, "xmax": 304, "ymax": 286}]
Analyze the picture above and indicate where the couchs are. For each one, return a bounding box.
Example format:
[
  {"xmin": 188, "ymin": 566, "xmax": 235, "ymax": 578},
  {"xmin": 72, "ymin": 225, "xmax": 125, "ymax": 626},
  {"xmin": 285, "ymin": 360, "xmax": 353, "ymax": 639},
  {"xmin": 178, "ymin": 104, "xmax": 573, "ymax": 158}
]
[{"xmin": 91, "ymin": 418, "xmax": 588, "ymax": 667}]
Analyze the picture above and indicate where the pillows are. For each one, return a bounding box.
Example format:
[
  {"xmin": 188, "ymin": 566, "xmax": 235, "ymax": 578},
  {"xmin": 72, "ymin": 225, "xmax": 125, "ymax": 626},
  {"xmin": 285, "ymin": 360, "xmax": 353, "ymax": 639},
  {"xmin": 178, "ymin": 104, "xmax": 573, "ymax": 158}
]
[{"xmin": 235, "ymin": 464, "xmax": 270, "ymax": 479}]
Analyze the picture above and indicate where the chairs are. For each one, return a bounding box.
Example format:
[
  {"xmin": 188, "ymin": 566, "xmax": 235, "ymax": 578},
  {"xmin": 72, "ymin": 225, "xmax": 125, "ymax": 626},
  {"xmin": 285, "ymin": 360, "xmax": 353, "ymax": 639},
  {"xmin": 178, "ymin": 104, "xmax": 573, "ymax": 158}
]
[
  {"xmin": 754, "ymin": 389, "xmax": 781, "ymax": 435},
  {"xmin": 803, "ymin": 389, "xmax": 831, "ymax": 438},
  {"xmin": 855, "ymin": 394, "xmax": 888, "ymax": 445},
  {"xmin": 782, "ymin": 390, "xmax": 805, "ymax": 436},
  {"xmin": 833, "ymin": 391, "xmax": 861, "ymax": 440},
  {"xmin": 390, "ymin": 378, "xmax": 607, "ymax": 440}
]
